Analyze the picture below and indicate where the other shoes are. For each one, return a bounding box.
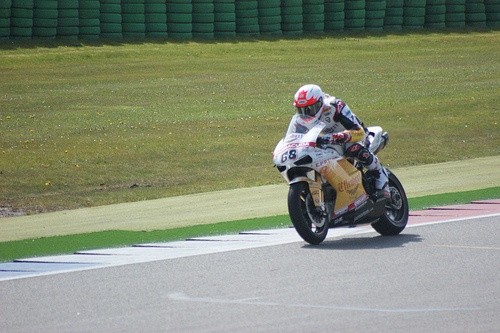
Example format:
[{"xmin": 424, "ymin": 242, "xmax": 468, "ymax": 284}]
[{"xmin": 375, "ymin": 165, "xmax": 391, "ymax": 199}]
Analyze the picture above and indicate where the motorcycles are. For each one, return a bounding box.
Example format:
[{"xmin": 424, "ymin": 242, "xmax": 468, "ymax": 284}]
[{"xmin": 269, "ymin": 114, "xmax": 410, "ymax": 242}]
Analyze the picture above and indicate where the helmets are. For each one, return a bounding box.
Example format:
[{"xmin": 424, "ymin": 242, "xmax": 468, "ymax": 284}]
[{"xmin": 293, "ymin": 84, "xmax": 325, "ymax": 126}]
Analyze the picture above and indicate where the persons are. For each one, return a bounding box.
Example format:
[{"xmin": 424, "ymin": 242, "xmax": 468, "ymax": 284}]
[{"xmin": 285, "ymin": 84, "xmax": 390, "ymax": 205}]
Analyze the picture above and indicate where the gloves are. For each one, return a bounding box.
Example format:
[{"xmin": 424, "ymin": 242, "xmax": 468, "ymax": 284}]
[{"xmin": 334, "ymin": 132, "xmax": 352, "ymax": 144}]
